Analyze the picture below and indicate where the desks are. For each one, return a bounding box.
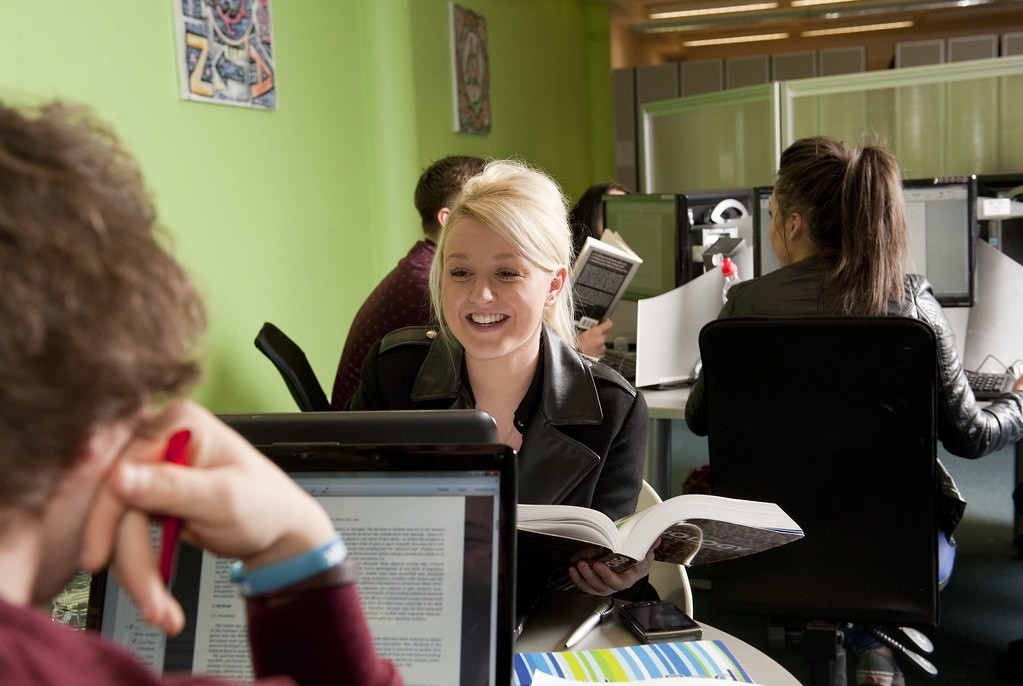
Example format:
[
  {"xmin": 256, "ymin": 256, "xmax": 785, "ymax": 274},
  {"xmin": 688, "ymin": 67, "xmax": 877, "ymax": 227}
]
[
  {"xmin": 636, "ymin": 383, "xmax": 1023, "ymax": 557},
  {"xmin": 512, "ymin": 606, "xmax": 801, "ymax": 685}
]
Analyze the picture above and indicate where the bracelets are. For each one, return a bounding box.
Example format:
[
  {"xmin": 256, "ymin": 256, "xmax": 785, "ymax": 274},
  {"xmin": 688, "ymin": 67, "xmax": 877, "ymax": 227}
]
[{"xmin": 227, "ymin": 532, "xmax": 346, "ymax": 597}]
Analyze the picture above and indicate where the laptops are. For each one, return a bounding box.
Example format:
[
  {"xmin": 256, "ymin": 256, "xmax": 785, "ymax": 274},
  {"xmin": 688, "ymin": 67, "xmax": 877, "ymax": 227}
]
[{"xmin": 92, "ymin": 409, "xmax": 527, "ymax": 684}]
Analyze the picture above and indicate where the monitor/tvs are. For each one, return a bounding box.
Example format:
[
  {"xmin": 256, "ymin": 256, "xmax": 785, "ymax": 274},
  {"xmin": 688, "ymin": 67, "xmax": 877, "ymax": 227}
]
[{"xmin": 601, "ymin": 172, "xmax": 1023, "ymax": 309}]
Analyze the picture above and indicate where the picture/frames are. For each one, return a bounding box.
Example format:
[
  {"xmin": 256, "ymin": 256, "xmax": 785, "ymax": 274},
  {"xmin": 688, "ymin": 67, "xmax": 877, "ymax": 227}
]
[
  {"xmin": 447, "ymin": 2, "xmax": 490, "ymax": 134},
  {"xmin": 175, "ymin": 0, "xmax": 278, "ymax": 111}
]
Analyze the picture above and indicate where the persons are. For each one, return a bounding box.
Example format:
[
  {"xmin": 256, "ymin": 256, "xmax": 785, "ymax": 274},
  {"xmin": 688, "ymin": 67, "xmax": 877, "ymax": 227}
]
[
  {"xmin": 567, "ymin": 183, "xmax": 630, "ymax": 258},
  {"xmin": 685, "ymin": 139, "xmax": 1023, "ymax": 686},
  {"xmin": 348, "ymin": 161, "xmax": 647, "ymax": 620},
  {"xmin": 0, "ymin": 97, "xmax": 402, "ymax": 686},
  {"xmin": 331, "ymin": 154, "xmax": 613, "ymax": 414}
]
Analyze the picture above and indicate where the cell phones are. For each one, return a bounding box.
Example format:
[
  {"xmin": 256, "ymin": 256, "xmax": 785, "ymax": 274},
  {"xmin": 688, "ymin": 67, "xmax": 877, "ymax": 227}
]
[{"xmin": 619, "ymin": 601, "xmax": 702, "ymax": 645}]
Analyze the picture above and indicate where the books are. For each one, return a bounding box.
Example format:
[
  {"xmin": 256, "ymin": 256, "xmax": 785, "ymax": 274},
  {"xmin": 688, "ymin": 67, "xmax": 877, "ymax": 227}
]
[
  {"xmin": 516, "ymin": 494, "xmax": 804, "ymax": 593},
  {"xmin": 565, "ymin": 229, "xmax": 642, "ymax": 328}
]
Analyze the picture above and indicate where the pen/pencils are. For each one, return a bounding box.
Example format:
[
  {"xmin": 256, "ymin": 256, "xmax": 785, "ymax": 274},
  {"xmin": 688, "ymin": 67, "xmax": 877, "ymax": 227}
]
[
  {"xmin": 565, "ymin": 596, "xmax": 615, "ymax": 648},
  {"xmin": 159, "ymin": 428, "xmax": 191, "ymax": 582}
]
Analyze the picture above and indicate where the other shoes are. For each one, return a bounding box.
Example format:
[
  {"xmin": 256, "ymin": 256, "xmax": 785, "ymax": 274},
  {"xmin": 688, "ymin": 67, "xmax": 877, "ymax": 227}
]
[{"xmin": 854, "ymin": 650, "xmax": 906, "ymax": 686}]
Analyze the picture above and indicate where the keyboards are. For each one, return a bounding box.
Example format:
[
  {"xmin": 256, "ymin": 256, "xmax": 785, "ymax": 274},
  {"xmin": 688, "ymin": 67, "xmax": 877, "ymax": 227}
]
[
  {"xmin": 600, "ymin": 352, "xmax": 637, "ymax": 381},
  {"xmin": 963, "ymin": 369, "xmax": 1016, "ymax": 400}
]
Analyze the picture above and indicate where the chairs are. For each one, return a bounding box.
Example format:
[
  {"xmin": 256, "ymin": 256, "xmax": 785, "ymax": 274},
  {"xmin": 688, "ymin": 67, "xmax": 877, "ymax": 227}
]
[
  {"xmin": 697, "ymin": 314, "xmax": 941, "ymax": 686},
  {"xmin": 253, "ymin": 323, "xmax": 334, "ymax": 413}
]
